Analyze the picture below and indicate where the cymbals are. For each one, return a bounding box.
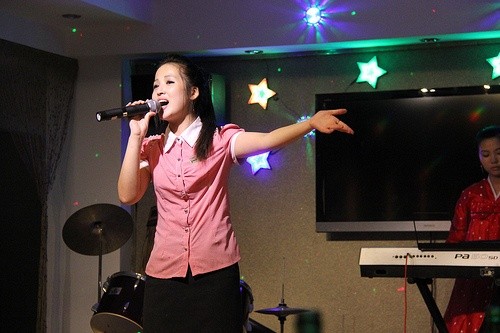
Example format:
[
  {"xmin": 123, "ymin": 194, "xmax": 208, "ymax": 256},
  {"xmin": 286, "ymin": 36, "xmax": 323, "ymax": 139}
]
[
  {"xmin": 61, "ymin": 201, "xmax": 136, "ymax": 257},
  {"xmin": 254, "ymin": 306, "xmax": 311, "ymax": 317}
]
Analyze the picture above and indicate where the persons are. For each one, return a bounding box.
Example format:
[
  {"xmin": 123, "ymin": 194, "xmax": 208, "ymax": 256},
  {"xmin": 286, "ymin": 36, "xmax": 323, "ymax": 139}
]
[
  {"xmin": 118, "ymin": 56, "xmax": 354, "ymax": 333},
  {"xmin": 443, "ymin": 125, "xmax": 500, "ymax": 333}
]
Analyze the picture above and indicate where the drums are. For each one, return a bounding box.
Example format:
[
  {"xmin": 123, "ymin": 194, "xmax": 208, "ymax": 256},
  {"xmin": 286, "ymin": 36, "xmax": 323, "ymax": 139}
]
[
  {"xmin": 239, "ymin": 276, "xmax": 254, "ymax": 333},
  {"xmin": 90, "ymin": 270, "xmax": 147, "ymax": 333}
]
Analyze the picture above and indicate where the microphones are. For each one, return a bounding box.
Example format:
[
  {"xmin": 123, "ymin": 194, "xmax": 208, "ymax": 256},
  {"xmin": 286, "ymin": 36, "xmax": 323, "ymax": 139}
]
[{"xmin": 96, "ymin": 100, "xmax": 161, "ymax": 122}]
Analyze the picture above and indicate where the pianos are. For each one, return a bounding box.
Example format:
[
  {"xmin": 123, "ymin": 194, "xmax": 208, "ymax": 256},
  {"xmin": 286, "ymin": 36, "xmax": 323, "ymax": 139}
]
[{"xmin": 358, "ymin": 239, "xmax": 500, "ymax": 333}]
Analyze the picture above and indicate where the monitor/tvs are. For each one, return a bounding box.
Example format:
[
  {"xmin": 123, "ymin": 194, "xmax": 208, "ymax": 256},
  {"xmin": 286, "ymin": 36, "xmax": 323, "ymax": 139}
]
[{"xmin": 314, "ymin": 85, "xmax": 499, "ymax": 235}]
